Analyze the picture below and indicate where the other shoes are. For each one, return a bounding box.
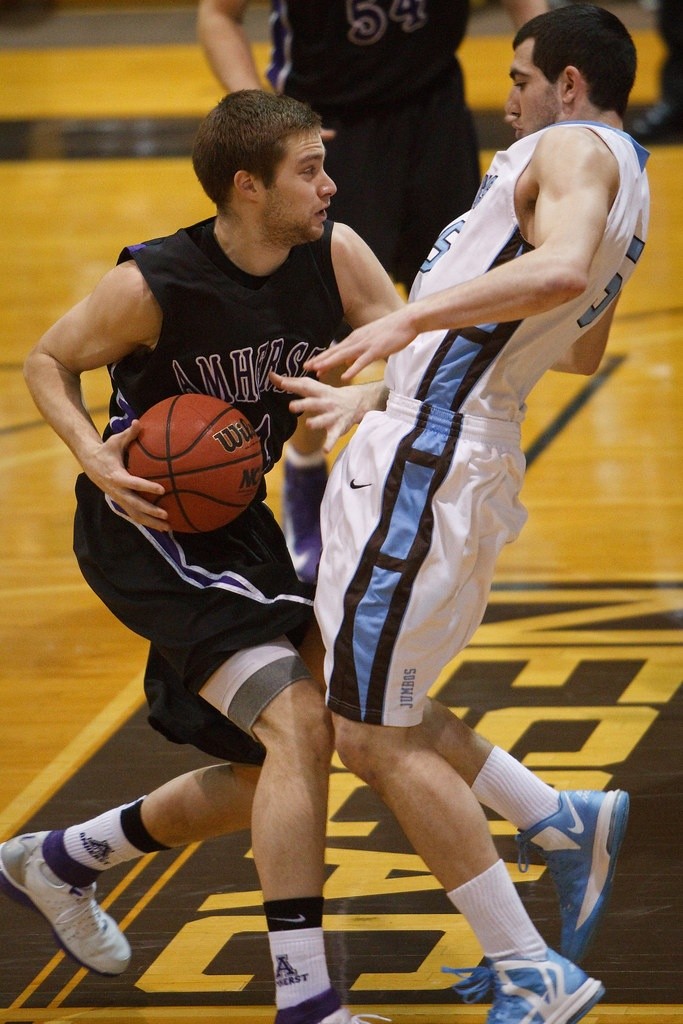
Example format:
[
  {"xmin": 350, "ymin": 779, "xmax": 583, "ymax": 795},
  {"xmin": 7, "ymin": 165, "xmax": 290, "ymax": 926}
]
[{"xmin": 625, "ymin": 102, "xmax": 683, "ymax": 144}]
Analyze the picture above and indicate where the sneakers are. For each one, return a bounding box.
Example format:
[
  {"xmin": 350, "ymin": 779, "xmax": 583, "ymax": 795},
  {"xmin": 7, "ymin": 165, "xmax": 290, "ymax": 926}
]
[
  {"xmin": 440, "ymin": 946, "xmax": 606, "ymax": 1024},
  {"xmin": 318, "ymin": 1007, "xmax": 392, "ymax": 1024},
  {"xmin": 512, "ymin": 788, "xmax": 629, "ymax": 963},
  {"xmin": 0, "ymin": 830, "xmax": 132, "ymax": 976},
  {"xmin": 281, "ymin": 461, "xmax": 329, "ymax": 588}
]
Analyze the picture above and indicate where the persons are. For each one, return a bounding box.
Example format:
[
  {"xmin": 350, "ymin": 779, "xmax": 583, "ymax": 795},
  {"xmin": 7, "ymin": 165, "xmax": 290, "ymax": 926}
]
[
  {"xmin": 195, "ymin": 0, "xmax": 683, "ymax": 583},
  {"xmin": 305, "ymin": 5, "xmax": 650, "ymax": 1024},
  {"xmin": 0, "ymin": 89, "xmax": 407, "ymax": 1024}
]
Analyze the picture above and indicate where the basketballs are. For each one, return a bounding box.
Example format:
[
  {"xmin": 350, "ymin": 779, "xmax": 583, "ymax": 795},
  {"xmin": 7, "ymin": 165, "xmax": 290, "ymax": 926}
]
[{"xmin": 124, "ymin": 390, "xmax": 268, "ymax": 537}]
